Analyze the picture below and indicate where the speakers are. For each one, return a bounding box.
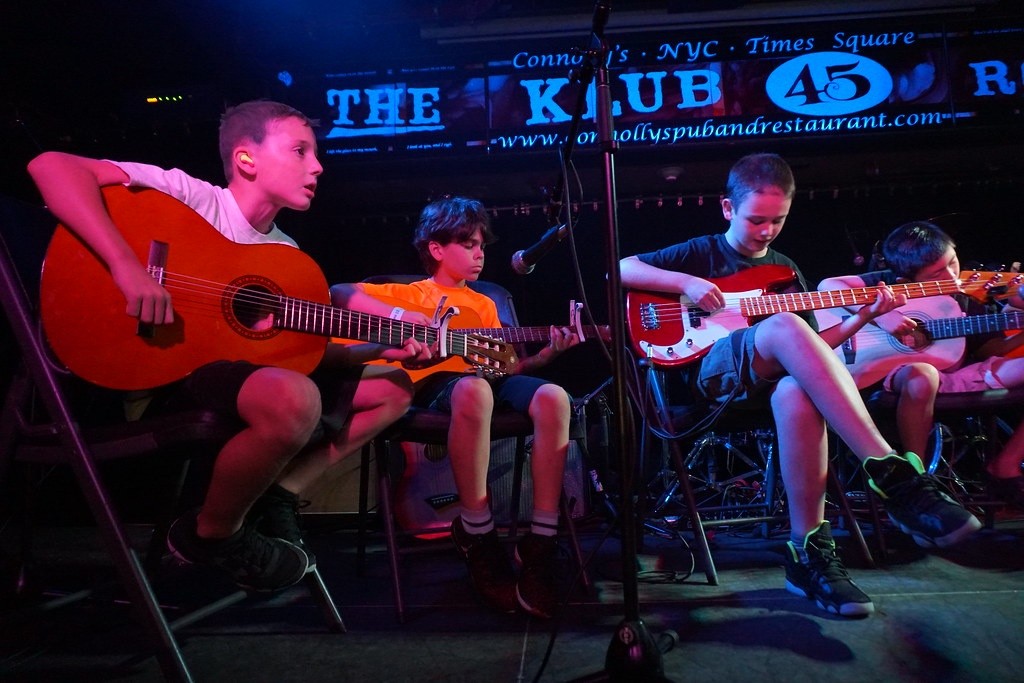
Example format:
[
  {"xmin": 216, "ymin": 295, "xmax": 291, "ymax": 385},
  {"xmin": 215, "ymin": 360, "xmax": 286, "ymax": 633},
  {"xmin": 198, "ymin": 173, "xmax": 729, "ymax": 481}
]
[
  {"xmin": 381, "ymin": 398, "xmax": 592, "ymax": 540},
  {"xmin": 272, "ymin": 437, "xmax": 378, "ymax": 520}
]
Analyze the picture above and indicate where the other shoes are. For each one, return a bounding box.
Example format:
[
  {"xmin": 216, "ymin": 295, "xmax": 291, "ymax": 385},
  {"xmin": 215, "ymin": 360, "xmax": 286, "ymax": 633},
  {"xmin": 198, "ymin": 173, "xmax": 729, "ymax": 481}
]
[{"xmin": 983, "ymin": 462, "xmax": 1024, "ymax": 510}]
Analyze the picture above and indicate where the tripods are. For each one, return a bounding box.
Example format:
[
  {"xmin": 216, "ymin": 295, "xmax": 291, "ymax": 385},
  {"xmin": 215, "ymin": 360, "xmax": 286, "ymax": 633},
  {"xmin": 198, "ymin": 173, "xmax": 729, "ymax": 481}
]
[
  {"xmin": 649, "ymin": 423, "xmax": 788, "ymax": 518},
  {"xmin": 540, "ymin": 0, "xmax": 681, "ymax": 682}
]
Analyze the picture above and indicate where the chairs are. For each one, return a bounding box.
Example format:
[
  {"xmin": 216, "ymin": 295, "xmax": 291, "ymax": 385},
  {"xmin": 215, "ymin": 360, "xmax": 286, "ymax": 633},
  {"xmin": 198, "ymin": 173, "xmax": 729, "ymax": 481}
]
[
  {"xmin": 359, "ymin": 406, "xmax": 592, "ymax": 615},
  {"xmin": 642, "ymin": 351, "xmax": 874, "ymax": 584},
  {"xmin": 832, "ymin": 383, "xmax": 1024, "ymax": 562},
  {"xmin": 1, "ymin": 230, "xmax": 348, "ymax": 682}
]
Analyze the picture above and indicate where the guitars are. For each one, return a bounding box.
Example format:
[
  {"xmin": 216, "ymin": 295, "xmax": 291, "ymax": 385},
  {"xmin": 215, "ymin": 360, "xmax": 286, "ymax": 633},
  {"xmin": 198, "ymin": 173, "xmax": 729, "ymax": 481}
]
[
  {"xmin": 36, "ymin": 181, "xmax": 522, "ymax": 396},
  {"xmin": 624, "ymin": 263, "xmax": 1024, "ymax": 368},
  {"xmin": 327, "ymin": 280, "xmax": 612, "ymax": 383},
  {"xmin": 815, "ymin": 293, "xmax": 1024, "ymax": 391}
]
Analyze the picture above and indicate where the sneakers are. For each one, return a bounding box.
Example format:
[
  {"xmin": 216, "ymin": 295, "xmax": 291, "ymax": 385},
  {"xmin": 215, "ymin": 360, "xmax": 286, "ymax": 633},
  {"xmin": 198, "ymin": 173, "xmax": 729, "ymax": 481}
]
[
  {"xmin": 450, "ymin": 515, "xmax": 519, "ymax": 616},
  {"xmin": 514, "ymin": 532, "xmax": 571, "ymax": 617},
  {"xmin": 166, "ymin": 512, "xmax": 309, "ymax": 592},
  {"xmin": 863, "ymin": 451, "xmax": 982, "ymax": 549},
  {"xmin": 244, "ymin": 491, "xmax": 316, "ymax": 573},
  {"xmin": 784, "ymin": 520, "xmax": 875, "ymax": 616}
]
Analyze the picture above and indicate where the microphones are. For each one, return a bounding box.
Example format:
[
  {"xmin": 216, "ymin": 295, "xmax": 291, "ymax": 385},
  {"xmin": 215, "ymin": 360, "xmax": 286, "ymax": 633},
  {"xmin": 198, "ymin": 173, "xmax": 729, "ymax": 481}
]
[
  {"xmin": 523, "ymin": 439, "xmax": 532, "ymax": 462},
  {"xmin": 511, "ymin": 214, "xmax": 577, "ymax": 275}
]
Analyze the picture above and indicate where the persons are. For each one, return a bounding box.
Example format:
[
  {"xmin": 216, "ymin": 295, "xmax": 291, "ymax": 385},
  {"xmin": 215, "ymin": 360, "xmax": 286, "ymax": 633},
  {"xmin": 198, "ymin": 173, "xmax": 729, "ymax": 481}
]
[
  {"xmin": 24, "ymin": 99, "xmax": 438, "ymax": 589},
  {"xmin": 604, "ymin": 154, "xmax": 982, "ymax": 615},
  {"xmin": 327, "ymin": 198, "xmax": 580, "ymax": 621},
  {"xmin": 813, "ymin": 220, "xmax": 1024, "ymax": 482}
]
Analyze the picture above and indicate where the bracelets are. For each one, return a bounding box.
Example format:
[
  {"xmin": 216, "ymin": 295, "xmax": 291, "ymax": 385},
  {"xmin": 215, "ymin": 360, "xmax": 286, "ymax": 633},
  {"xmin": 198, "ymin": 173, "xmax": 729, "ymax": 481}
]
[{"xmin": 389, "ymin": 307, "xmax": 405, "ymax": 320}]
